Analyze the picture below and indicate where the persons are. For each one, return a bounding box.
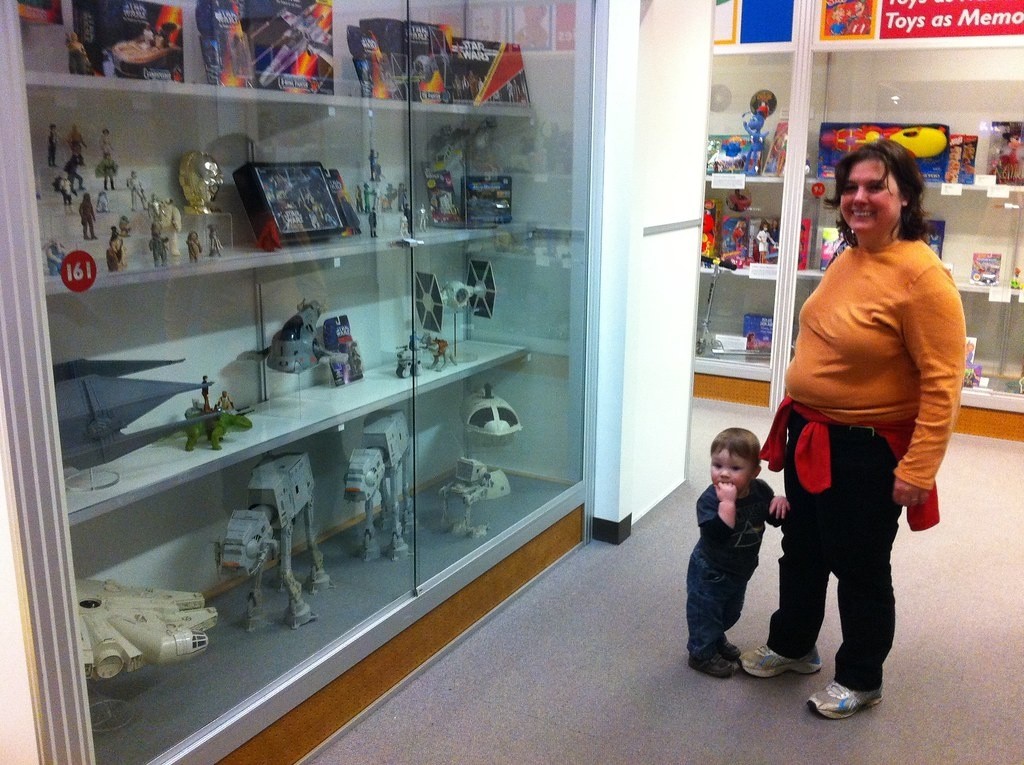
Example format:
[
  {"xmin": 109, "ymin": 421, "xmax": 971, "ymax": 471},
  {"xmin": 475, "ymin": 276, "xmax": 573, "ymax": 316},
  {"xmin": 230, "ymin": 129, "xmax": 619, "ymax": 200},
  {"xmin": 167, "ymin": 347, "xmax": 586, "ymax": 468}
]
[
  {"xmin": 686, "ymin": 428, "xmax": 790, "ymax": 677},
  {"xmin": 742, "ymin": 106, "xmax": 770, "ymax": 176},
  {"xmin": 47, "ymin": 122, "xmax": 225, "ymax": 275},
  {"xmin": 355, "ymin": 149, "xmax": 429, "ymax": 238},
  {"xmin": 201, "ymin": 376, "xmax": 235, "ymax": 413},
  {"xmin": 738, "ymin": 138, "xmax": 966, "ymax": 719},
  {"xmin": 757, "ymin": 220, "xmax": 777, "ymax": 264},
  {"xmin": 66, "ymin": 30, "xmax": 91, "ymax": 74}
]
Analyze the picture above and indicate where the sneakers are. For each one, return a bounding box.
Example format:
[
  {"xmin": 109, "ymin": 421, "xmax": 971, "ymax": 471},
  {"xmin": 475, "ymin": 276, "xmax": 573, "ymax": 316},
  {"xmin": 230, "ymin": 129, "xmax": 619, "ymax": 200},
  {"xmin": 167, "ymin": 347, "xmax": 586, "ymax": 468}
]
[
  {"xmin": 720, "ymin": 642, "xmax": 741, "ymax": 662},
  {"xmin": 806, "ymin": 680, "xmax": 883, "ymax": 719},
  {"xmin": 688, "ymin": 653, "xmax": 734, "ymax": 677},
  {"xmin": 737, "ymin": 644, "xmax": 822, "ymax": 677}
]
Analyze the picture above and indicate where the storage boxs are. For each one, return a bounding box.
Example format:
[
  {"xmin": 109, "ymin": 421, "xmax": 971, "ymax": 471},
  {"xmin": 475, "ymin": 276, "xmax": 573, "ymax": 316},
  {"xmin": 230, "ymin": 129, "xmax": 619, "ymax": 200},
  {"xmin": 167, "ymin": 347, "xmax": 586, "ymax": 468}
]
[
  {"xmin": 232, "ymin": 160, "xmax": 346, "ymax": 247},
  {"xmin": 701, "ymin": 198, "xmax": 724, "ymax": 259},
  {"xmin": 945, "ymin": 134, "xmax": 979, "ymax": 184}
]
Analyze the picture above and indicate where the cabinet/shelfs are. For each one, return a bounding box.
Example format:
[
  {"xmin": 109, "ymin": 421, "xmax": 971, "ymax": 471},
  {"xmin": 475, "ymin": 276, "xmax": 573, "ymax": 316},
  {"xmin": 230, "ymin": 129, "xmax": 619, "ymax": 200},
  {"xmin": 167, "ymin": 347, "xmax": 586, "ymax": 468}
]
[
  {"xmin": 0, "ymin": 0, "xmax": 609, "ymax": 765},
  {"xmin": 693, "ymin": 0, "xmax": 1024, "ymax": 442}
]
